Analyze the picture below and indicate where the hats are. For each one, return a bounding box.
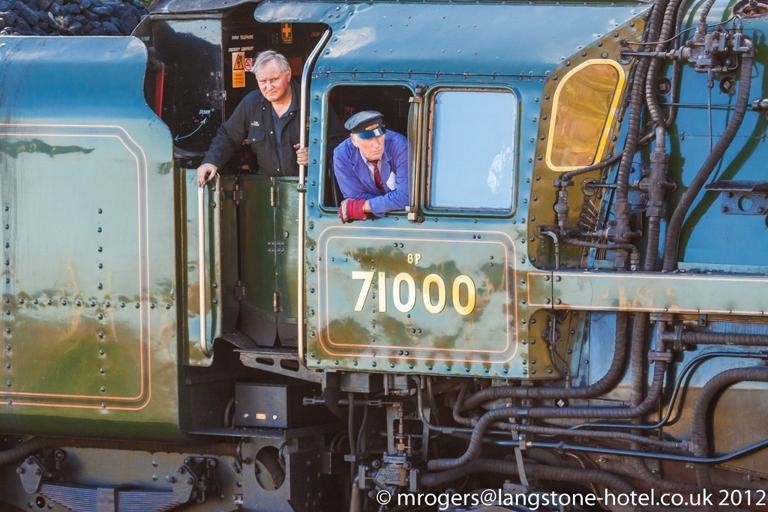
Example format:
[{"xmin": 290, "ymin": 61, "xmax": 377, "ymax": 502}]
[{"xmin": 344, "ymin": 110, "xmax": 386, "ymax": 139}]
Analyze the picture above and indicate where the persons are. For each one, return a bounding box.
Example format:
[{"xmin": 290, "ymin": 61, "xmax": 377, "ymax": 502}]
[
  {"xmin": 331, "ymin": 109, "xmax": 410, "ymax": 224},
  {"xmin": 196, "ymin": 49, "xmax": 309, "ymax": 188}
]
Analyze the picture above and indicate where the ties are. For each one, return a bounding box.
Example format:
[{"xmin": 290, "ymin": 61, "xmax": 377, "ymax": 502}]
[{"xmin": 368, "ymin": 159, "xmax": 386, "ymax": 195}]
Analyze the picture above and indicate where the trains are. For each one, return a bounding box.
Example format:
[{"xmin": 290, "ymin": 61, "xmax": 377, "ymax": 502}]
[{"xmin": 0, "ymin": 1, "xmax": 768, "ymax": 512}]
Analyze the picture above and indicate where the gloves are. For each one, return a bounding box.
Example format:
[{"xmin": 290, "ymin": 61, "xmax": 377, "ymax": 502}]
[{"xmin": 337, "ymin": 198, "xmax": 368, "ymax": 224}]
